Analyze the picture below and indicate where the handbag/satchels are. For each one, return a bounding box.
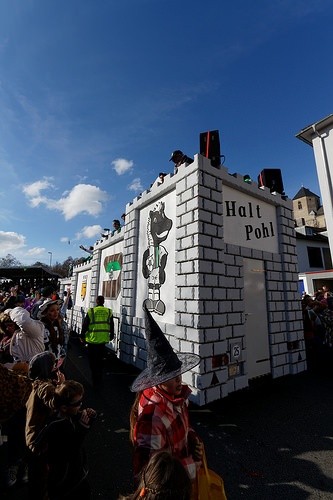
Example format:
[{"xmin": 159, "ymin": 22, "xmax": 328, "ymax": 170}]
[{"xmin": 192, "ymin": 449, "xmax": 226, "ymax": 500}]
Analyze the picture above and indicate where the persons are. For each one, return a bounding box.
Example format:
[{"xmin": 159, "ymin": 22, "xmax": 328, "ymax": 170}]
[
  {"xmin": 79, "ymin": 245, "xmax": 94, "ymax": 254},
  {"xmin": 118, "ymin": 450, "xmax": 192, "ymax": 500},
  {"xmin": 81, "ymin": 295, "xmax": 115, "ymax": 372},
  {"xmin": 0, "ymin": 279, "xmax": 98, "ymax": 500},
  {"xmin": 301, "ymin": 283, "xmax": 333, "ymax": 348},
  {"xmin": 82, "ymin": 246, "xmax": 93, "ymax": 263},
  {"xmin": 69, "ymin": 259, "xmax": 80, "ymax": 277},
  {"xmin": 168, "ymin": 150, "xmax": 194, "ymax": 174},
  {"xmin": 244, "ymin": 174, "xmax": 252, "ymax": 182},
  {"xmin": 100, "ymin": 220, "xmax": 123, "ymax": 239},
  {"xmin": 128, "ymin": 308, "xmax": 204, "ymax": 500},
  {"xmin": 121, "ymin": 214, "xmax": 126, "ymax": 221},
  {"xmin": 158, "ymin": 172, "xmax": 167, "ymax": 187}
]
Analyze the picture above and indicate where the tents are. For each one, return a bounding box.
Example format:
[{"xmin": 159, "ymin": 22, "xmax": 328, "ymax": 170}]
[{"xmin": 0, "ymin": 265, "xmax": 63, "ymax": 279}]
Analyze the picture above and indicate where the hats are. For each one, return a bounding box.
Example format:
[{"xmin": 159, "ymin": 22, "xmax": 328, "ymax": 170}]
[
  {"xmin": 28, "ymin": 351, "xmax": 64, "ymax": 380},
  {"xmin": 37, "ymin": 294, "xmax": 64, "ymax": 319},
  {"xmin": 131, "ymin": 303, "xmax": 202, "ymax": 393}
]
